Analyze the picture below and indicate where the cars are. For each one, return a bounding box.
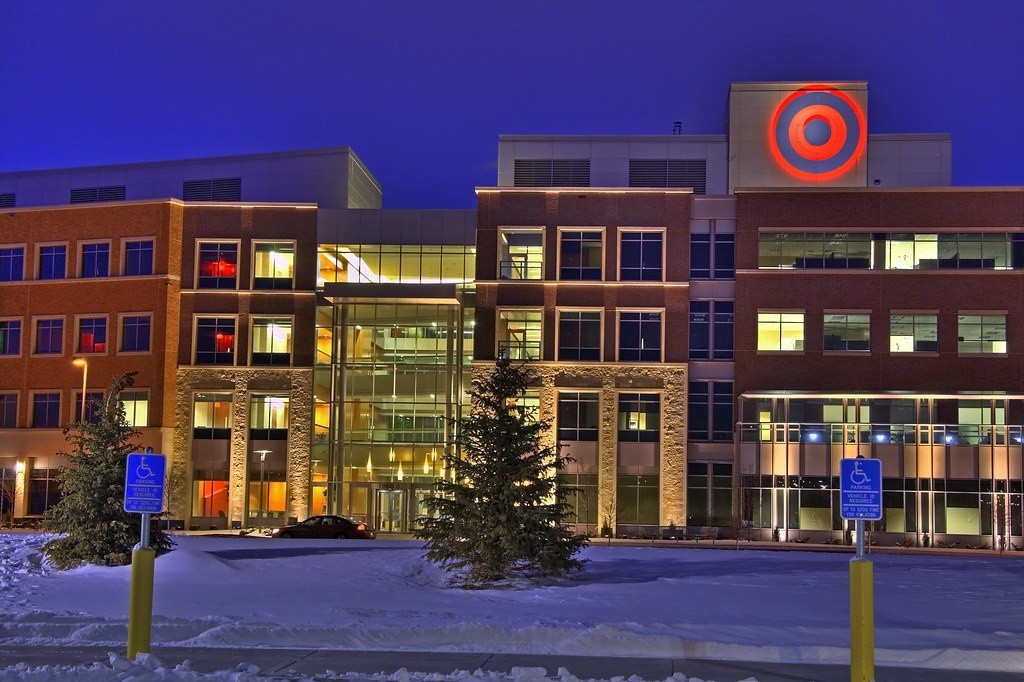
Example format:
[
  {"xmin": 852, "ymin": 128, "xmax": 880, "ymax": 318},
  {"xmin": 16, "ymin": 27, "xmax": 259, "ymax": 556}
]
[{"xmin": 272, "ymin": 515, "xmax": 377, "ymax": 539}]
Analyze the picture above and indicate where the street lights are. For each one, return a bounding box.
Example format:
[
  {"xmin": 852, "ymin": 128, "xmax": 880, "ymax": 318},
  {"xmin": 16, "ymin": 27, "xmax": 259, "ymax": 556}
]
[{"xmin": 73, "ymin": 358, "xmax": 88, "ymax": 452}]
